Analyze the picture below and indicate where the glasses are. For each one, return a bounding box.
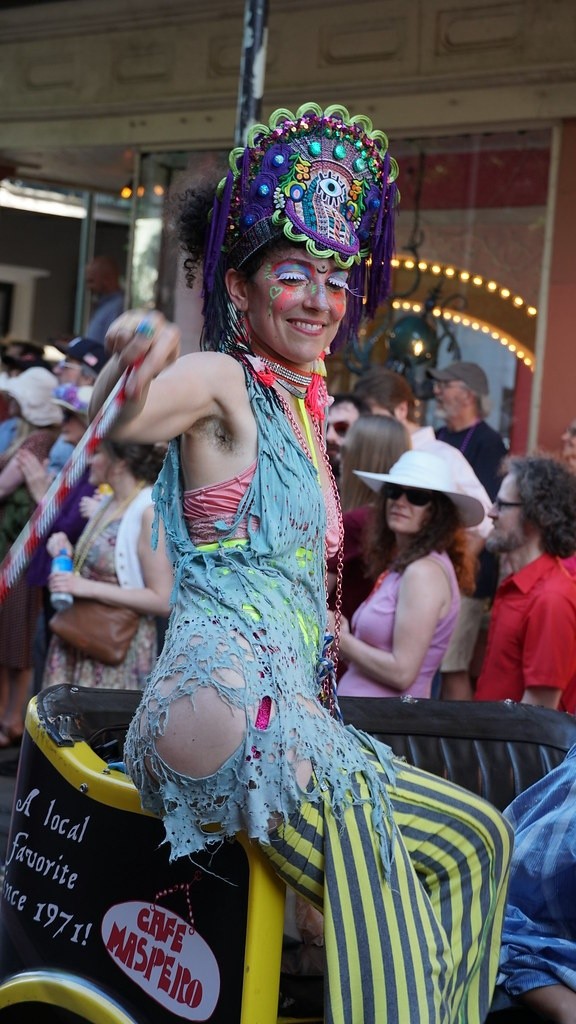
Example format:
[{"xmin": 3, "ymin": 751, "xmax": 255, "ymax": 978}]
[
  {"xmin": 496, "ymin": 498, "xmax": 523, "ymax": 511},
  {"xmin": 61, "ymin": 360, "xmax": 84, "ymax": 371},
  {"xmin": 327, "ymin": 421, "xmax": 350, "ymax": 436},
  {"xmin": 435, "ymin": 381, "xmax": 465, "ymax": 390},
  {"xmin": 384, "ymin": 483, "xmax": 435, "ymax": 506},
  {"xmin": 63, "ymin": 411, "xmax": 77, "ymax": 423}
]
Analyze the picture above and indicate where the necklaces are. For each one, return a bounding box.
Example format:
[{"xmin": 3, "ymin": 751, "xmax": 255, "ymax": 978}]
[
  {"xmin": 251, "ymin": 349, "xmax": 344, "ymax": 720},
  {"xmin": 73, "ymin": 477, "xmax": 145, "ymax": 574},
  {"xmin": 437, "ymin": 419, "xmax": 478, "ymax": 452}
]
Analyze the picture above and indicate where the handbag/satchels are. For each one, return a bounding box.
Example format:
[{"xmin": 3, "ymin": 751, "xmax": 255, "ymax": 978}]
[{"xmin": 48, "ymin": 574, "xmax": 141, "ymax": 664}]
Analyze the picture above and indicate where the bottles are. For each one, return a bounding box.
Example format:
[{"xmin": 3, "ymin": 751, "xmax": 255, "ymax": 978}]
[{"xmin": 50, "ymin": 547, "xmax": 75, "ymax": 609}]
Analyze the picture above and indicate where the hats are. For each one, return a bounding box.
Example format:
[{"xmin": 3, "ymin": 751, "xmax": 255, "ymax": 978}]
[
  {"xmin": 54, "ymin": 335, "xmax": 110, "ymax": 373},
  {"xmin": 352, "ymin": 447, "xmax": 485, "ymax": 527},
  {"xmin": 51, "ymin": 385, "xmax": 95, "ymax": 425},
  {"xmin": 202, "ymin": 103, "xmax": 401, "ymax": 352},
  {"xmin": 426, "ymin": 362, "xmax": 489, "ymax": 397}
]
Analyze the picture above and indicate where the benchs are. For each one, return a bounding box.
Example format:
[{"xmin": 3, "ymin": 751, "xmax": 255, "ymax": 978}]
[{"xmin": 0, "ymin": 685, "xmax": 576, "ymax": 1024}]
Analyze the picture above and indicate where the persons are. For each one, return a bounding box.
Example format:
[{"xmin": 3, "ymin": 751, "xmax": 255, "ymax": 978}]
[
  {"xmin": 0, "ymin": 237, "xmax": 176, "ymax": 747},
  {"xmin": 322, "ymin": 361, "xmax": 576, "ymax": 791},
  {"xmin": 490, "ymin": 740, "xmax": 576, "ymax": 1024},
  {"xmin": 89, "ymin": 102, "xmax": 516, "ymax": 1024}
]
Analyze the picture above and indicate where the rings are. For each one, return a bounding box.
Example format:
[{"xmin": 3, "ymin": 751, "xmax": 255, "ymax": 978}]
[{"xmin": 135, "ymin": 321, "xmax": 154, "ymax": 338}]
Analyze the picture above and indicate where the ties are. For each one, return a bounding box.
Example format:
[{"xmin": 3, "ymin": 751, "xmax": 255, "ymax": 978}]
[{"xmin": 0, "ymin": 367, "xmax": 63, "ymax": 427}]
